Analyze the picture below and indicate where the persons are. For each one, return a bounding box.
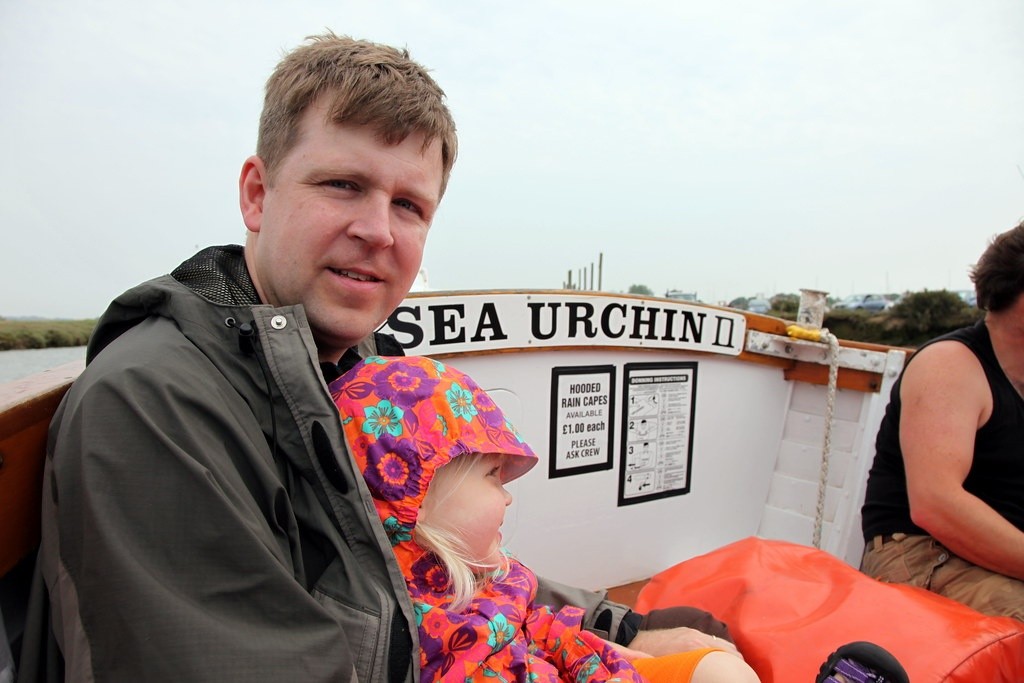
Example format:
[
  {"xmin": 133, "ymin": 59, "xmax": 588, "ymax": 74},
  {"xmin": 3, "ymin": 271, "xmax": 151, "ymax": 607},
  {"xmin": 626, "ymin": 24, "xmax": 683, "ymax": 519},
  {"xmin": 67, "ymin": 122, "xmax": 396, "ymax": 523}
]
[
  {"xmin": 329, "ymin": 354, "xmax": 909, "ymax": 682},
  {"xmin": 20, "ymin": 30, "xmax": 746, "ymax": 683},
  {"xmin": 862, "ymin": 221, "xmax": 1024, "ymax": 621}
]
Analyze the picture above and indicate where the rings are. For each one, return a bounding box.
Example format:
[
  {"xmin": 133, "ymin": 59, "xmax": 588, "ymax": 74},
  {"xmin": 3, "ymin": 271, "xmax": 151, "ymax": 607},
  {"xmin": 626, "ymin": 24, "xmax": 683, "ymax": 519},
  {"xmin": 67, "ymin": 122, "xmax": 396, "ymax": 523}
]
[{"xmin": 710, "ymin": 633, "xmax": 717, "ymax": 640}]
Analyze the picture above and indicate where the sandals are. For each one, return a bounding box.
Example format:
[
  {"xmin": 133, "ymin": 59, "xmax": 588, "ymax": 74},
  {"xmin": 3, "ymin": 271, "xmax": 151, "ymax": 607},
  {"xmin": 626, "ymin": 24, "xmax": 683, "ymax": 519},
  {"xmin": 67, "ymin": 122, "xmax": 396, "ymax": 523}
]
[{"xmin": 815, "ymin": 641, "xmax": 911, "ymax": 683}]
